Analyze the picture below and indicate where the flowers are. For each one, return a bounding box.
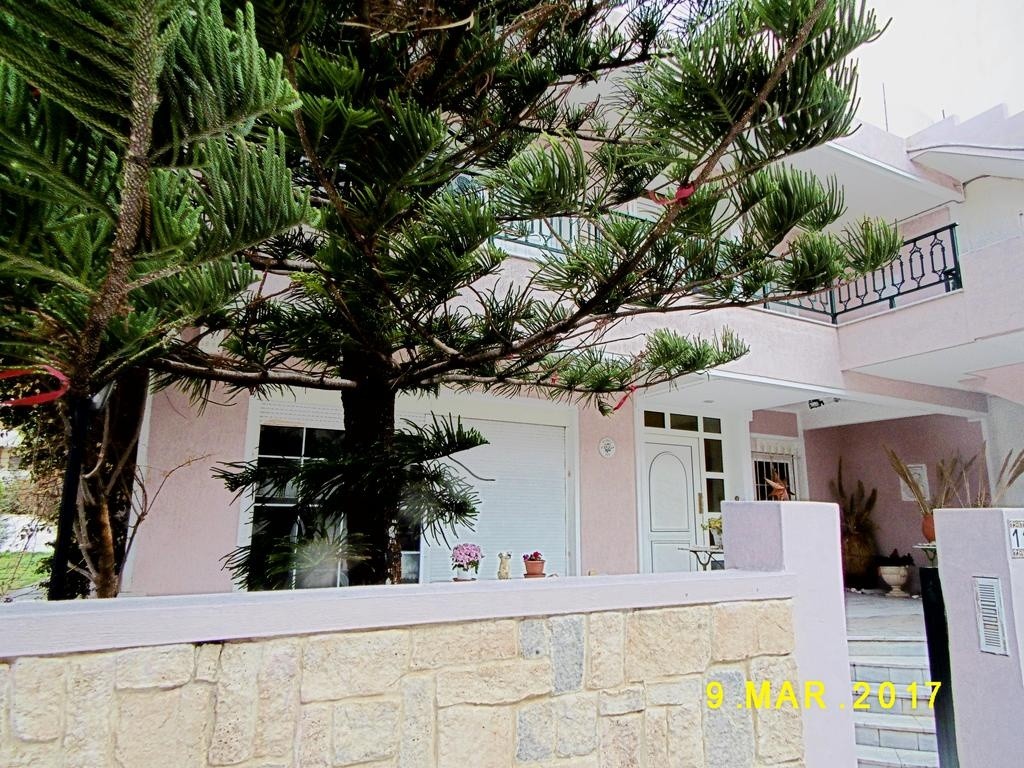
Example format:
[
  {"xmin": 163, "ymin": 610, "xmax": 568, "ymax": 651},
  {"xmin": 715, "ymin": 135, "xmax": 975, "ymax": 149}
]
[
  {"xmin": 523, "ymin": 551, "xmax": 543, "ymax": 560},
  {"xmin": 498, "ymin": 551, "xmax": 512, "ymax": 559},
  {"xmin": 451, "ymin": 543, "xmax": 485, "ymax": 574}
]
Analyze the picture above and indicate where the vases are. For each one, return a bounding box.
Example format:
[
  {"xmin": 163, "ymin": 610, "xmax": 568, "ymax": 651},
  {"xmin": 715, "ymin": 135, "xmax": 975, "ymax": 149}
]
[
  {"xmin": 454, "ymin": 565, "xmax": 477, "ymax": 582},
  {"xmin": 836, "ymin": 456, "xmax": 876, "ymax": 584},
  {"xmin": 498, "ymin": 558, "xmax": 513, "ymax": 579},
  {"xmin": 922, "ymin": 514, "xmax": 935, "ymax": 544},
  {"xmin": 524, "ymin": 560, "xmax": 546, "ymax": 578}
]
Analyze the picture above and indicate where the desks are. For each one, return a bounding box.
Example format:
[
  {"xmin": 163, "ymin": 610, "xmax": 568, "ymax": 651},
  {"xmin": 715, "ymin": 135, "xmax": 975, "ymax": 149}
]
[{"xmin": 677, "ymin": 546, "xmax": 724, "ymax": 571}]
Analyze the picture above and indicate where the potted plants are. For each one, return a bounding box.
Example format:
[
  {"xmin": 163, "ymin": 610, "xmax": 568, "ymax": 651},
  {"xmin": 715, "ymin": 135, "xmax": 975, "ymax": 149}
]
[
  {"xmin": 701, "ymin": 515, "xmax": 723, "ymax": 546},
  {"xmin": 876, "ymin": 549, "xmax": 915, "ymax": 594}
]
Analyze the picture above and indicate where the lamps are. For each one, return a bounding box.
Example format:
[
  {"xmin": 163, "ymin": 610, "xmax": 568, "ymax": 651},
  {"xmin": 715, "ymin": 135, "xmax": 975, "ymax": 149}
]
[{"xmin": 809, "ymin": 397, "xmax": 840, "ymax": 409}]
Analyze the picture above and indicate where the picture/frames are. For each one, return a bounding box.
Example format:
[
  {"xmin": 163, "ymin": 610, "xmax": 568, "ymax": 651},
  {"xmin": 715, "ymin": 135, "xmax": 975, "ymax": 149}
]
[{"xmin": 899, "ymin": 464, "xmax": 930, "ymax": 501}]
